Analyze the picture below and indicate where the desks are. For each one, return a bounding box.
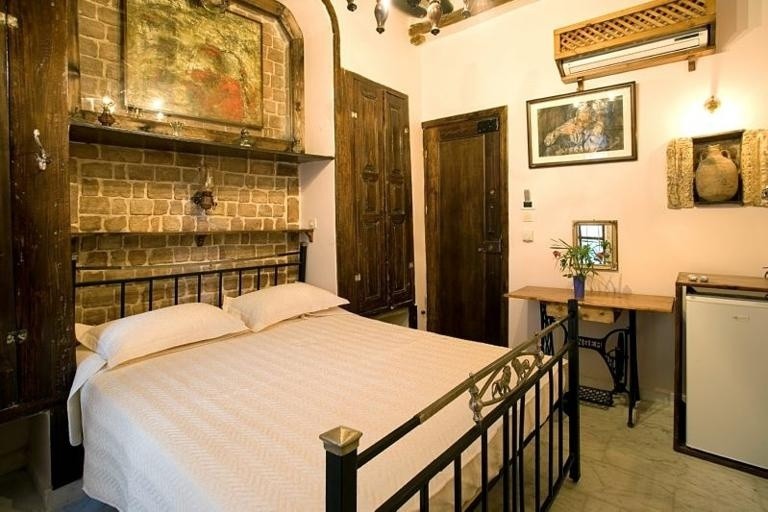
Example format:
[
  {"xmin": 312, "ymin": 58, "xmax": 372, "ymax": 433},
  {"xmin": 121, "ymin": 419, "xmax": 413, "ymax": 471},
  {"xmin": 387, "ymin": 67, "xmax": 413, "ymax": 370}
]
[{"xmin": 501, "ymin": 284, "xmax": 675, "ymax": 429}]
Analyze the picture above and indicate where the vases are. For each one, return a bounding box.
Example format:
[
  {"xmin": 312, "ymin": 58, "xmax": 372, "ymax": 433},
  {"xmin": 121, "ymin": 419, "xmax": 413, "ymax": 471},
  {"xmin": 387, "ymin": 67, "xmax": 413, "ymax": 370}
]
[
  {"xmin": 571, "ymin": 274, "xmax": 586, "ymax": 301},
  {"xmin": 692, "ymin": 144, "xmax": 741, "ymax": 204}
]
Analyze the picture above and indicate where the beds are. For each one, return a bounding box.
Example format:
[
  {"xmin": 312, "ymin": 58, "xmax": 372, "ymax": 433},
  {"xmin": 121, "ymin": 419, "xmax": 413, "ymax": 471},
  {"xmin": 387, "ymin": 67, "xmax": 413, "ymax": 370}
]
[{"xmin": 68, "ymin": 239, "xmax": 583, "ymax": 511}]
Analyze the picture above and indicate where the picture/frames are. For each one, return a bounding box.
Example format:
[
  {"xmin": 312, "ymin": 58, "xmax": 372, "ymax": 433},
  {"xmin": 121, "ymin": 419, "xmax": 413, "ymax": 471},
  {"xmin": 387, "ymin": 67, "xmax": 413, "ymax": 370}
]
[
  {"xmin": 117, "ymin": 0, "xmax": 267, "ymax": 134},
  {"xmin": 525, "ymin": 80, "xmax": 639, "ymax": 174}
]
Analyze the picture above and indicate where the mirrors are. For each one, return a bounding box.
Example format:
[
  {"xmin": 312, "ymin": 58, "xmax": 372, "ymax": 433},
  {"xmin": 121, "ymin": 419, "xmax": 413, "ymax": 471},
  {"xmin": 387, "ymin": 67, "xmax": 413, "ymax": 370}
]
[{"xmin": 571, "ymin": 218, "xmax": 618, "ymax": 273}]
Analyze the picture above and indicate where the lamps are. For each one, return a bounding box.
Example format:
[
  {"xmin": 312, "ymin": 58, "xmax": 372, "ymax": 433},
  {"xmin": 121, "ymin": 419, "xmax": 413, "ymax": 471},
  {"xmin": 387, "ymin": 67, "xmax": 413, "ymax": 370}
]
[{"xmin": 344, "ymin": 1, "xmax": 476, "ymax": 37}]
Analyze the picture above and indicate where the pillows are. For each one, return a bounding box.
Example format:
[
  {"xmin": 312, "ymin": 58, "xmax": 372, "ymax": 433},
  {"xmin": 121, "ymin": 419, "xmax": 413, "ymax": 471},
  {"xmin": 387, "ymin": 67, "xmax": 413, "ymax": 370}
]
[
  {"xmin": 221, "ymin": 281, "xmax": 352, "ymax": 333},
  {"xmin": 74, "ymin": 299, "xmax": 251, "ymax": 371}
]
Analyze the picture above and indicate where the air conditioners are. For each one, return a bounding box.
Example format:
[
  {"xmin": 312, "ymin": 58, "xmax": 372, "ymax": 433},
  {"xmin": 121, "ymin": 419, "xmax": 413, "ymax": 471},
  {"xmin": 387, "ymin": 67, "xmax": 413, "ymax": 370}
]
[{"xmin": 552, "ymin": 2, "xmax": 720, "ymax": 93}]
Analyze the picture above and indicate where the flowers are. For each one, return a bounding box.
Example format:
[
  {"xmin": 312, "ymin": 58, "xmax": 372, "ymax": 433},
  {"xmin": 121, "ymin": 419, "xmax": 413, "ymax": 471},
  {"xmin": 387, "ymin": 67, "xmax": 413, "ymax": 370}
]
[
  {"xmin": 547, "ymin": 236, "xmax": 599, "ymax": 280},
  {"xmin": 590, "ymin": 239, "xmax": 612, "ymax": 266}
]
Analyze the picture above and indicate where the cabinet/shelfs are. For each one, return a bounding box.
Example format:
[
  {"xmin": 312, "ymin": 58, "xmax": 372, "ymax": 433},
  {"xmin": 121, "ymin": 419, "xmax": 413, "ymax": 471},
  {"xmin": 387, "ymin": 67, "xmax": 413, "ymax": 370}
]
[
  {"xmin": 666, "ymin": 269, "xmax": 767, "ymax": 482},
  {"xmin": 1, "ymin": 0, "xmax": 75, "ymax": 424},
  {"xmin": 335, "ymin": 67, "xmax": 418, "ymax": 319},
  {"xmin": 68, "ymin": 117, "xmax": 335, "ymax": 254}
]
